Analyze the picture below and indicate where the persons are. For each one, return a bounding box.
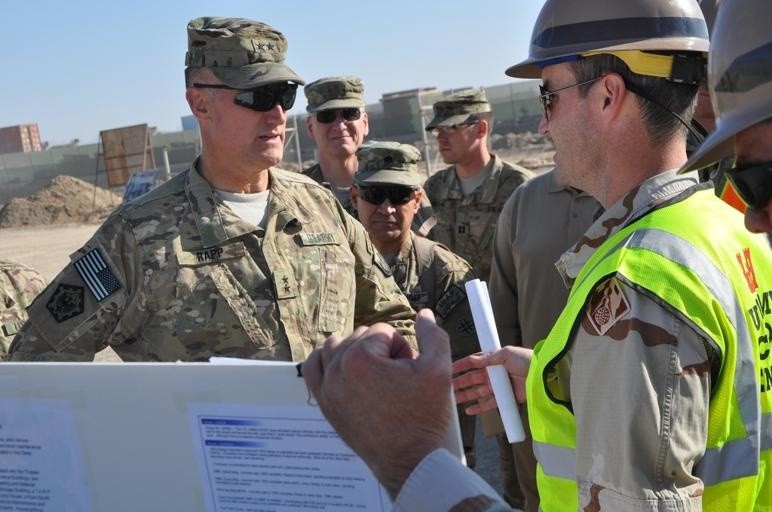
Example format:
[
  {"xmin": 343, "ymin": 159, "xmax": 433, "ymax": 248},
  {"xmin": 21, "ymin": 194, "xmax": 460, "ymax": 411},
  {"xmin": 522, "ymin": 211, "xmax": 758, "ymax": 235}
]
[
  {"xmin": 2, "ymin": 15, "xmax": 421, "ymax": 363},
  {"xmin": 296, "ymin": 2, "xmax": 771, "ymax": 511},
  {"xmin": 1, "ymin": 260, "xmax": 48, "ymax": 362},
  {"xmin": 685, "ymin": 1, "xmax": 748, "ymax": 217},
  {"xmin": 485, "ymin": 169, "xmax": 605, "ymax": 511},
  {"xmin": 347, "ymin": 140, "xmax": 473, "ymax": 325},
  {"xmin": 673, "ymin": 2, "xmax": 772, "ymax": 250},
  {"xmin": 423, "ymin": 85, "xmax": 536, "ymax": 287},
  {"xmin": 299, "ymin": 75, "xmax": 370, "ymax": 222}
]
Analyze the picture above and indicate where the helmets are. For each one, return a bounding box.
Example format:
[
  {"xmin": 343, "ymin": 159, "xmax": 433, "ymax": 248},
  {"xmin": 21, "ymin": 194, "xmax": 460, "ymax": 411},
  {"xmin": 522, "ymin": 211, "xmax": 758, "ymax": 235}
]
[
  {"xmin": 676, "ymin": 0, "xmax": 772, "ymax": 176},
  {"xmin": 505, "ymin": 1, "xmax": 710, "ymax": 80}
]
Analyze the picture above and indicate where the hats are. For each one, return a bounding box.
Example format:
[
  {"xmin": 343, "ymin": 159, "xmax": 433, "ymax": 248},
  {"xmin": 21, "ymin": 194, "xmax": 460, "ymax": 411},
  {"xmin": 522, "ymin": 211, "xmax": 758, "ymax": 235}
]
[
  {"xmin": 353, "ymin": 140, "xmax": 421, "ymax": 187},
  {"xmin": 184, "ymin": 16, "xmax": 305, "ymax": 90},
  {"xmin": 303, "ymin": 75, "xmax": 365, "ymax": 112},
  {"xmin": 425, "ymin": 87, "xmax": 493, "ymax": 132}
]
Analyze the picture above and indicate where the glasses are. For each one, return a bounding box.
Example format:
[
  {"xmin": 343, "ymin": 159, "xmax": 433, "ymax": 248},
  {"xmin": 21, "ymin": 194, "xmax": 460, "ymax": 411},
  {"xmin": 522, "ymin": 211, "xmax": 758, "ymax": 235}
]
[
  {"xmin": 432, "ymin": 117, "xmax": 480, "ymax": 136},
  {"xmin": 538, "ymin": 75, "xmax": 602, "ymax": 121},
  {"xmin": 193, "ymin": 80, "xmax": 298, "ymax": 112},
  {"xmin": 720, "ymin": 155, "xmax": 772, "ymax": 209},
  {"xmin": 358, "ymin": 186, "xmax": 416, "ymax": 205}
]
